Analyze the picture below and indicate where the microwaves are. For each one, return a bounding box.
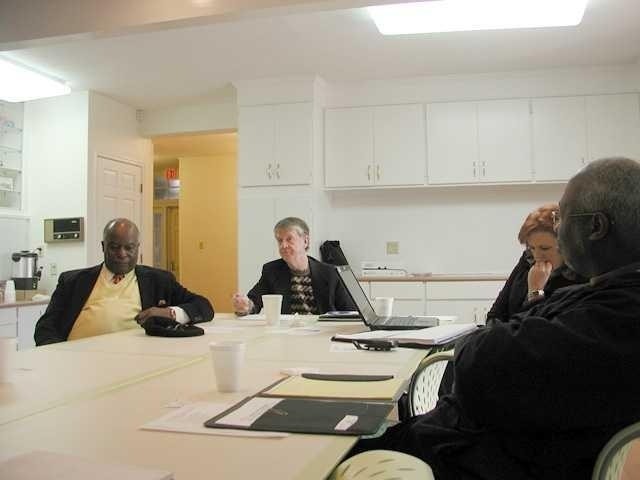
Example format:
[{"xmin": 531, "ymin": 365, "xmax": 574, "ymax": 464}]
[{"xmin": 42, "ymin": 217, "xmax": 84, "ymax": 243}]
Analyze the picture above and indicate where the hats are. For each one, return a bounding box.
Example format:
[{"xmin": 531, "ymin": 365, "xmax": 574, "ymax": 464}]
[{"xmin": 141, "ymin": 316, "xmax": 204, "ymax": 337}]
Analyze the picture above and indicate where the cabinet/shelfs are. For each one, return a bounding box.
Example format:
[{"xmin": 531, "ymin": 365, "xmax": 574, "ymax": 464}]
[
  {"xmin": 240, "ymin": 100, "xmax": 313, "ymax": 188},
  {"xmin": 425, "ymin": 279, "xmax": 507, "ymax": 323},
  {"xmin": 0, "ymin": 307, "xmax": 17, "ymax": 338},
  {"xmin": 0, "ymin": 123, "xmax": 23, "ymax": 210},
  {"xmin": 16, "ymin": 305, "xmax": 49, "ymax": 353},
  {"xmin": 359, "ymin": 280, "xmax": 425, "ymax": 320}
]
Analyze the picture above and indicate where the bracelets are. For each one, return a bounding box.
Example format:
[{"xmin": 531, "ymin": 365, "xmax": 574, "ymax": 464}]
[
  {"xmin": 168, "ymin": 309, "xmax": 178, "ymax": 321},
  {"xmin": 526, "ymin": 288, "xmax": 547, "ymax": 299}
]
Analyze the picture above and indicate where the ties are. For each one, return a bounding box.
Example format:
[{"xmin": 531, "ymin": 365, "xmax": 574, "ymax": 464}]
[{"xmin": 113, "ymin": 273, "xmax": 124, "ymax": 283}]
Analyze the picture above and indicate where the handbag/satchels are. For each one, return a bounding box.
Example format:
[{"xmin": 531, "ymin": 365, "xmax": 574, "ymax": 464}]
[{"xmin": 320, "ymin": 240, "xmax": 348, "ymax": 265}]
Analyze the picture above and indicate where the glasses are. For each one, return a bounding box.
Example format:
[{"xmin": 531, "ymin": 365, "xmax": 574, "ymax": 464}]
[{"xmin": 551, "ymin": 210, "xmax": 616, "ymax": 231}]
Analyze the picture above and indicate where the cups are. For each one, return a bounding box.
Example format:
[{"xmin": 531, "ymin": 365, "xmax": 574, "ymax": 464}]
[
  {"xmin": 261, "ymin": 294, "xmax": 283, "ymax": 324},
  {"xmin": 208, "ymin": 340, "xmax": 249, "ymax": 392},
  {"xmin": 4, "ymin": 280, "xmax": 17, "ymax": 304},
  {"xmin": 375, "ymin": 297, "xmax": 393, "ymax": 317}
]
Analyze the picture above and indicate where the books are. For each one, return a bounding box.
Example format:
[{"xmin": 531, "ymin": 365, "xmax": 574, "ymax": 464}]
[
  {"xmin": 317, "ymin": 310, "xmax": 362, "ymax": 321},
  {"xmin": 331, "ymin": 322, "xmax": 480, "ymax": 350}
]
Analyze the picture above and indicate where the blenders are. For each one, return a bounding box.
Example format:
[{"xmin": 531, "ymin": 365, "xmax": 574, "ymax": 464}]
[{"xmin": 12, "ymin": 249, "xmax": 38, "ymax": 300}]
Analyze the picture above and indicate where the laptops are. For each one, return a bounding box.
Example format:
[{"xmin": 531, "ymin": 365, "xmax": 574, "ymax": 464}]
[{"xmin": 335, "ymin": 265, "xmax": 439, "ymax": 330}]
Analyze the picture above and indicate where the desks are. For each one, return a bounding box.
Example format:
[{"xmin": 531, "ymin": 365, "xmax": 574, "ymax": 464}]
[{"xmin": 1, "ymin": 311, "xmax": 431, "ymax": 479}]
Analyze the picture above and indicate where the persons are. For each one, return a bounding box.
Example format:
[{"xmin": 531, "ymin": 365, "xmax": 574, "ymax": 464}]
[
  {"xmin": 340, "ymin": 154, "xmax": 638, "ymax": 480},
  {"xmin": 28, "ymin": 218, "xmax": 215, "ymax": 345},
  {"xmin": 230, "ymin": 213, "xmax": 360, "ymax": 316},
  {"xmin": 479, "ymin": 201, "xmax": 591, "ymax": 325}
]
